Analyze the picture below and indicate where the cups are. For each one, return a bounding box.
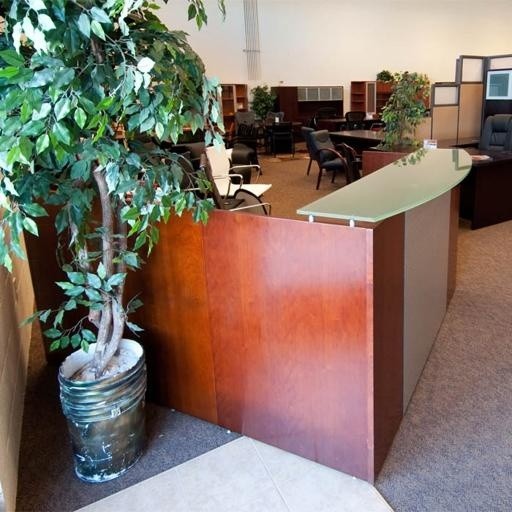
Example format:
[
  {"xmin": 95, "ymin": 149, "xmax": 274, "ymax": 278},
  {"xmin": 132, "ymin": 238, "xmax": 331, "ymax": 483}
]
[
  {"xmin": 365, "ymin": 112, "xmax": 374, "ymax": 120},
  {"xmin": 423, "ymin": 139, "xmax": 438, "ymax": 149},
  {"xmin": 274, "ymin": 116, "xmax": 280, "ymax": 123}
]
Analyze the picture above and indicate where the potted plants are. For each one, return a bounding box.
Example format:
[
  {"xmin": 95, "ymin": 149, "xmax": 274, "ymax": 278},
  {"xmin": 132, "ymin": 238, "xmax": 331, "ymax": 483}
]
[{"xmin": 0, "ymin": 0, "xmax": 226, "ymax": 483}]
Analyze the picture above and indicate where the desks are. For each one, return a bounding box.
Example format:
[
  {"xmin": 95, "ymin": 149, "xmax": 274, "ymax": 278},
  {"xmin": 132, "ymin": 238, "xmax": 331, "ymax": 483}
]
[{"xmin": 436, "ymin": 136, "xmax": 512, "ymax": 230}]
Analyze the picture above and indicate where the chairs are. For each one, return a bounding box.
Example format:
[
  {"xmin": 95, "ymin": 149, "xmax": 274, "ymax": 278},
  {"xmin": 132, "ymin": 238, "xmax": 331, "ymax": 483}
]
[
  {"xmin": 478, "ymin": 114, "xmax": 512, "ymax": 151},
  {"xmin": 127, "ymin": 110, "xmax": 387, "ymax": 218}
]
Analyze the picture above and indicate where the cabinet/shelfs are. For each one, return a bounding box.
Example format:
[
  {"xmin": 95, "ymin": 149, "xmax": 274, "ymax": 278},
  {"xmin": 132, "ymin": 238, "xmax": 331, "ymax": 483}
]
[
  {"xmin": 350, "ymin": 80, "xmax": 429, "ymax": 117},
  {"xmin": 219, "ymin": 84, "xmax": 249, "ymax": 147}
]
